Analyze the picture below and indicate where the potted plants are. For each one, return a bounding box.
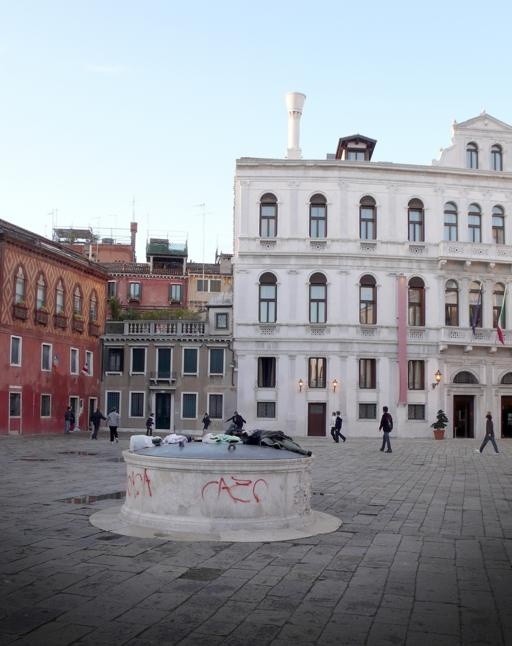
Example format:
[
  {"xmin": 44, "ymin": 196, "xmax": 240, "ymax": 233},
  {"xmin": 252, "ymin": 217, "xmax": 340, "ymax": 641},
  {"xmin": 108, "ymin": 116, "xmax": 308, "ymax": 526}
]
[
  {"xmin": 430, "ymin": 409, "xmax": 449, "ymax": 440},
  {"xmin": 109, "ymin": 299, "xmax": 194, "ymax": 321}
]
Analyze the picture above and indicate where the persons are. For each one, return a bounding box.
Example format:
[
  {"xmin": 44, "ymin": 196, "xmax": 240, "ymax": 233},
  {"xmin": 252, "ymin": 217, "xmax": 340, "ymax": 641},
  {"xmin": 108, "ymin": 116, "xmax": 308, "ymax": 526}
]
[
  {"xmin": 106, "ymin": 405, "xmax": 120, "ymax": 444},
  {"xmin": 146, "ymin": 413, "xmax": 155, "ymax": 437},
  {"xmin": 201, "ymin": 412, "xmax": 211, "ymax": 432},
  {"xmin": 378, "ymin": 406, "xmax": 395, "ymax": 453},
  {"xmin": 330, "ymin": 411, "xmax": 336, "ymax": 441},
  {"xmin": 224, "ymin": 409, "xmax": 246, "ymax": 431},
  {"xmin": 334, "ymin": 411, "xmax": 347, "ymax": 444},
  {"xmin": 474, "ymin": 414, "xmax": 500, "ymax": 455},
  {"xmin": 64, "ymin": 405, "xmax": 75, "ymax": 434},
  {"xmin": 90, "ymin": 407, "xmax": 106, "ymax": 441}
]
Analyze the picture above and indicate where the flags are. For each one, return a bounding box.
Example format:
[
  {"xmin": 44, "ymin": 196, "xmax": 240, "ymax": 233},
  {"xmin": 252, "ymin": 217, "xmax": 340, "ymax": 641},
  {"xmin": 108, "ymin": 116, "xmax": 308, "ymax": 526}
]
[
  {"xmin": 496, "ymin": 296, "xmax": 505, "ymax": 346},
  {"xmin": 471, "ymin": 287, "xmax": 482, "ymax": 336}
]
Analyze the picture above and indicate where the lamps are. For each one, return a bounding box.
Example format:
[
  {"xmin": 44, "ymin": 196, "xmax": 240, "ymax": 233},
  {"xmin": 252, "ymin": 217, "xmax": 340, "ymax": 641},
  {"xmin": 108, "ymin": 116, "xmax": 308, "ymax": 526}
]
[
  {"xmin": 332, "ymin": 379, "xmax": 337, "ymax": 392},
  {"xmin": 433, "ymin": 369, "xmax": 443, "ymax": 388},
  {"xmin": 299, "ymin": 378, "xmax": 303, "ymax": 392}
]
